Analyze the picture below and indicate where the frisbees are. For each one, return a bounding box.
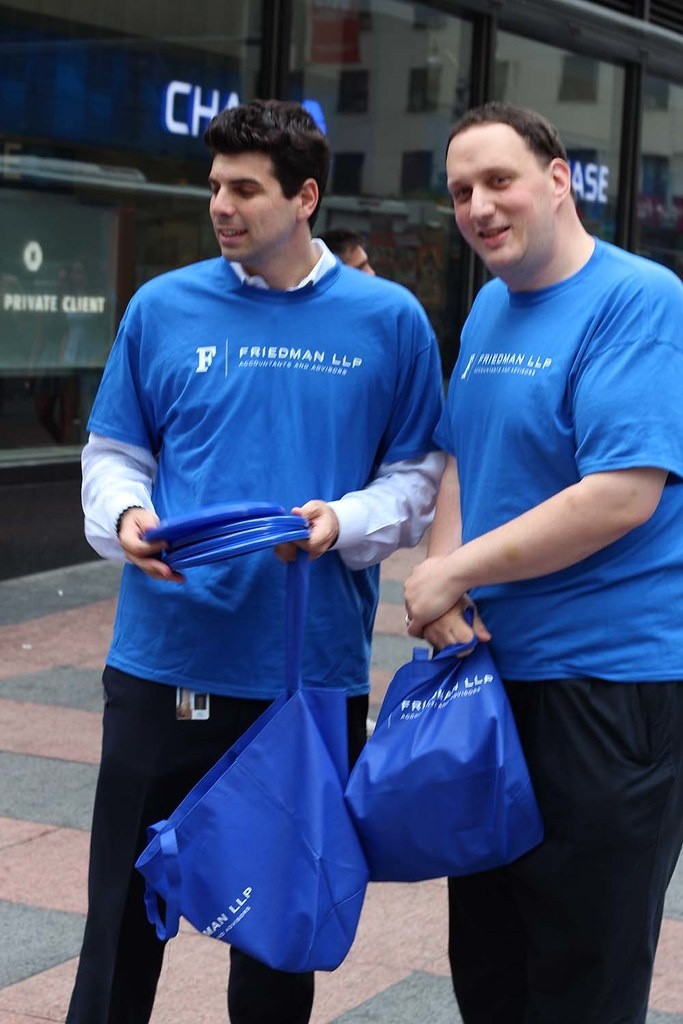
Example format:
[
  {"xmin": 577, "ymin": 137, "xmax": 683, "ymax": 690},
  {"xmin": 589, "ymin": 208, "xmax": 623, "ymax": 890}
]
[{"xmin": 137, "ymin": 501, "xmax": 312, "ymax": 570}]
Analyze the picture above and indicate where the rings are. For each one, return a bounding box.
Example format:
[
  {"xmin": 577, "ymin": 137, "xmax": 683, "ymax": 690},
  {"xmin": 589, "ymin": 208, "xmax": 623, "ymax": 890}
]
[{"xmin": 404, "ymin": 615, "xmax": 413, "ymax": 626}]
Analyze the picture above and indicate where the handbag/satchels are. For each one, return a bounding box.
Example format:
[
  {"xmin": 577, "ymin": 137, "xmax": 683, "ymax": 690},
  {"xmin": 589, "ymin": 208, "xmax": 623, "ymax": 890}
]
[
  {"xmin": 344, "ymin": 604, "xmax": 546, "ymax": 883},
  {"xmin": 134, "ymin": 539, "xmax": 368, "ymax": 973}
]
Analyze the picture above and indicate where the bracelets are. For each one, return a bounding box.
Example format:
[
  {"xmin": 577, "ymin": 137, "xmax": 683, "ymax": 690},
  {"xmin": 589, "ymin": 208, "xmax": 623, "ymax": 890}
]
[{"xmin": 116, "ymin": 505, "xmax": 145, "ymax": 540}]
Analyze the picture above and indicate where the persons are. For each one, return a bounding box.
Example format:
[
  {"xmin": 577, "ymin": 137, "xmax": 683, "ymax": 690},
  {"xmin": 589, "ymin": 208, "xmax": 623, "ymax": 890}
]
[
  {"xmin": 319, "ymin": 226, "xmax": 375, "ymax": 279},
  {"xmin": 62, "ymin": 99, "xmax": 451, "ymax": 1023},
  {"xmin": 399, "ymin": 95, "xmax": 683, "ymax": 1023}
]
